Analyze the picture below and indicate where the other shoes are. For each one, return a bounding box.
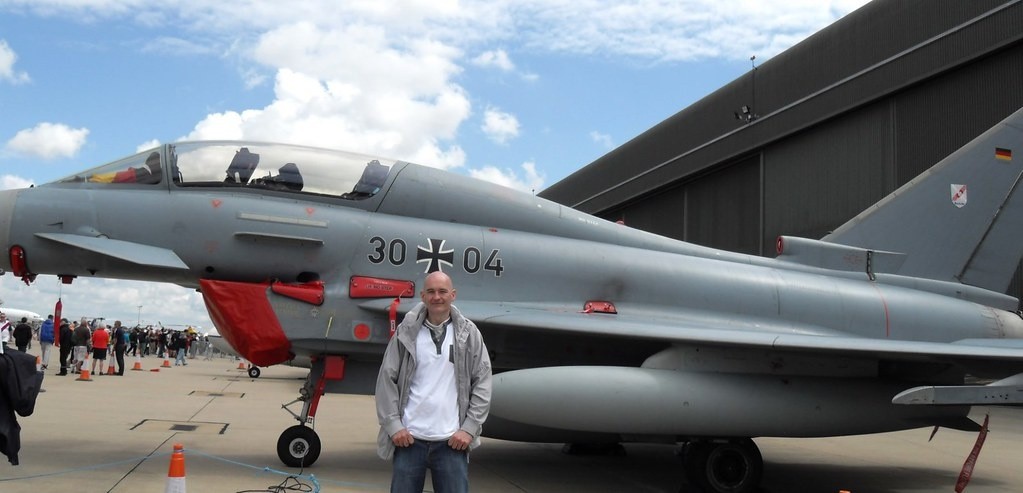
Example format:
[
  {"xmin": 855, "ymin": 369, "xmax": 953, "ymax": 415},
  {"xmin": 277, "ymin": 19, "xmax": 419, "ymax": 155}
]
[
  {"xmin": 91, "ymin": 372, "xmax": 95, "ymax": 374},
  {"xmin": 99, "ymin": 372, "xmax": 103, "ymax": 375},
  {"xmin": 56, "ymin": 373, "xmax": 65, "ymax": 376}
]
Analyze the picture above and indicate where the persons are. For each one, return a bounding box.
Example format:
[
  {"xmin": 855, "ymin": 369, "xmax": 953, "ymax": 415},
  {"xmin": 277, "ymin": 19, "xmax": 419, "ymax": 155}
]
[
  {"xmin": 375, "ymin": 271, "xmax": 493, "ymax": 493},
  {"xmin": 0, "ymin": 312, "xmax": 197, "ymax": 376}
]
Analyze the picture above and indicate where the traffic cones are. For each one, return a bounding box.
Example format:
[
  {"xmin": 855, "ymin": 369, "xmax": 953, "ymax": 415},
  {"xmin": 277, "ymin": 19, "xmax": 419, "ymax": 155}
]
[
  {"xmin": 165, "ymin": 444, "xmax": 186, "ymax": 493},
  {"xmin": 131, "ymin": 350, "xmax": 143, "ymax": 370},
  {"xmin": 160, "ymin": 349, "xmax": 171, "ymax": 367},
  {"xmin": 35, "ymin": 355, "xmax": 46, "ymax": 392},
  {"xmin": 104, "ymin": 350, "xmax": 117, "ymax": 375},
  {"xmin": 76, "ymin": 353, "xmax": 92, "ymax": 381}
]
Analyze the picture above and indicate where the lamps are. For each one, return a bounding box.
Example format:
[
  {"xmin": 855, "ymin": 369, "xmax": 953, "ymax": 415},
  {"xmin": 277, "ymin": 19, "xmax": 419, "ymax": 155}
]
[{"xmin": 733, "ymin": 104, "xmax": 756, "ymax": 125}]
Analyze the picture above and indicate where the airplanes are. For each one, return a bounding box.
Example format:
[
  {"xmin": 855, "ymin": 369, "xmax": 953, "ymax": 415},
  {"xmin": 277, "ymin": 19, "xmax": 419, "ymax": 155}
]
[
  {"xmin": 0, "ymin": 307, "xmax": 45, "ymax": 328},
  {"xmin": 205, "ymin": 325, "xmax": 312, "ymax": 378},
  {"xmin": 0, "ymin": 106, "xmax": 1023, "ymax": 493}
]
